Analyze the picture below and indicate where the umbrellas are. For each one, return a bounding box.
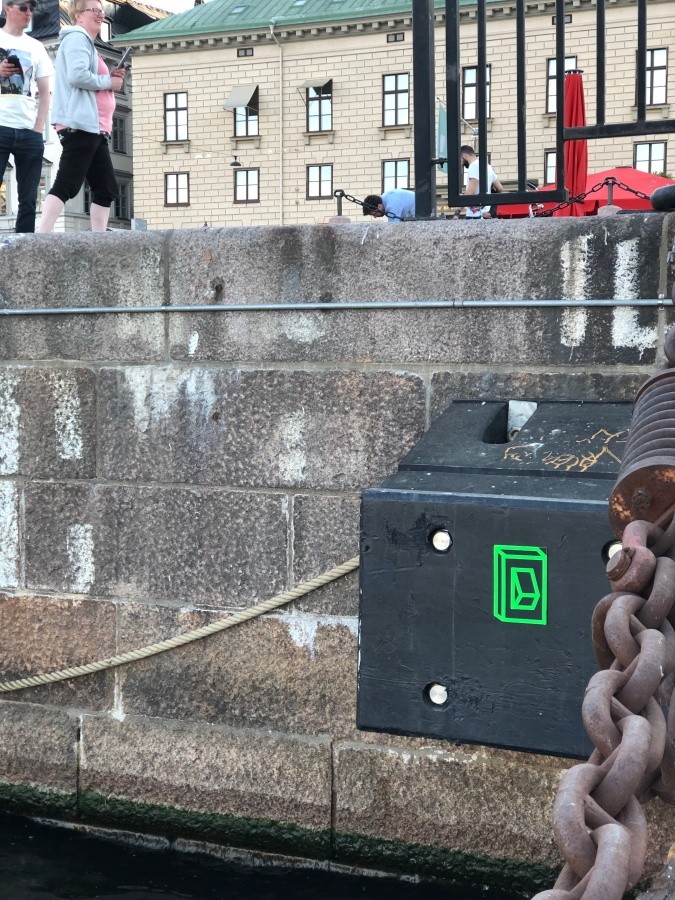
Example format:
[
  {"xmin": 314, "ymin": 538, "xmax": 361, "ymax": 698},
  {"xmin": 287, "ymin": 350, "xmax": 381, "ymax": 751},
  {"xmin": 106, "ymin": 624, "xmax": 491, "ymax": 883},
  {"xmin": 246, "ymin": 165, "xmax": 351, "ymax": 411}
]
[{"xmin": 563, "ymin": 70, "xmax": 585, "ymax": 217}]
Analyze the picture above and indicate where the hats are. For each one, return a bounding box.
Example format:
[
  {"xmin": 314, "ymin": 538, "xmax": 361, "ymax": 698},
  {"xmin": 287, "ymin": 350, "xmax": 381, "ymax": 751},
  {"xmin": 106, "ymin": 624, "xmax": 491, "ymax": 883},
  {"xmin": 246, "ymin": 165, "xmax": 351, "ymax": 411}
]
[{"xmin": 4, "ymin": 0, "xmax": 37, "ymax": 6}]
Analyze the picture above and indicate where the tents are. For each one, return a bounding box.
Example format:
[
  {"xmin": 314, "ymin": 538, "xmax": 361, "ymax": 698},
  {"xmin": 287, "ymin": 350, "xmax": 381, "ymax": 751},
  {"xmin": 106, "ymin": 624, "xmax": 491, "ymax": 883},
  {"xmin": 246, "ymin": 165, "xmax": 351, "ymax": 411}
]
[{"xmin": 495, "ymin": 167, "xmax": 675, "ymax": 217}]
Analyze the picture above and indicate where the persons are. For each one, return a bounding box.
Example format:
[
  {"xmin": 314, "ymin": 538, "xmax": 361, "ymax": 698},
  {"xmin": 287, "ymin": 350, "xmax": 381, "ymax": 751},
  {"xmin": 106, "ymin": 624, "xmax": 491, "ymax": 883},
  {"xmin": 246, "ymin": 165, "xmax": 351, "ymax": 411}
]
[
  {"xmin": 458, "ymin": 145, "xmax": 504, "ymax": 220},
  {"xmin": 37, "ymin": 0, "xmax": 127, "ymax": 231},
  {"xmin": 0, "ymin": 0, "xmax": 53, "ymax": 232},
  {"xmin": 362, "ymin": 188, "xmax": 417, "ymax": 222}
]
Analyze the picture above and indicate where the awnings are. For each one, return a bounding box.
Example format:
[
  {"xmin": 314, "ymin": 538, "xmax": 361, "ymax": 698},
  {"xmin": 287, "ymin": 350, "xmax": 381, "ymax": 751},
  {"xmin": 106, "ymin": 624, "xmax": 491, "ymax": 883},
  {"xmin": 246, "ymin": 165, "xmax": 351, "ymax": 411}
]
[
  {"xmin": 296, "ymin": 78, "xmax": 332, "ymax": 105},
  {"xmin": 222, "ymin": 84, "xmax": 260, "ymax": 114}
]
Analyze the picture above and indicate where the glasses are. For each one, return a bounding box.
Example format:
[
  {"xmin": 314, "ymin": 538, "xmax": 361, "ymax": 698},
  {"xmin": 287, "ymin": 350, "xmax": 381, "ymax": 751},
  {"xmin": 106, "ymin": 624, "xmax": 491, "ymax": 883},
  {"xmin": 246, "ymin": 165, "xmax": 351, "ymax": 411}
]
[
  {"xmin": 79, "ymin": 7, "xmax": 106, "ymax": 17},
  {"xmin": 9, "ymin": 6, "xmax": 34, "ymax": 12}
]
